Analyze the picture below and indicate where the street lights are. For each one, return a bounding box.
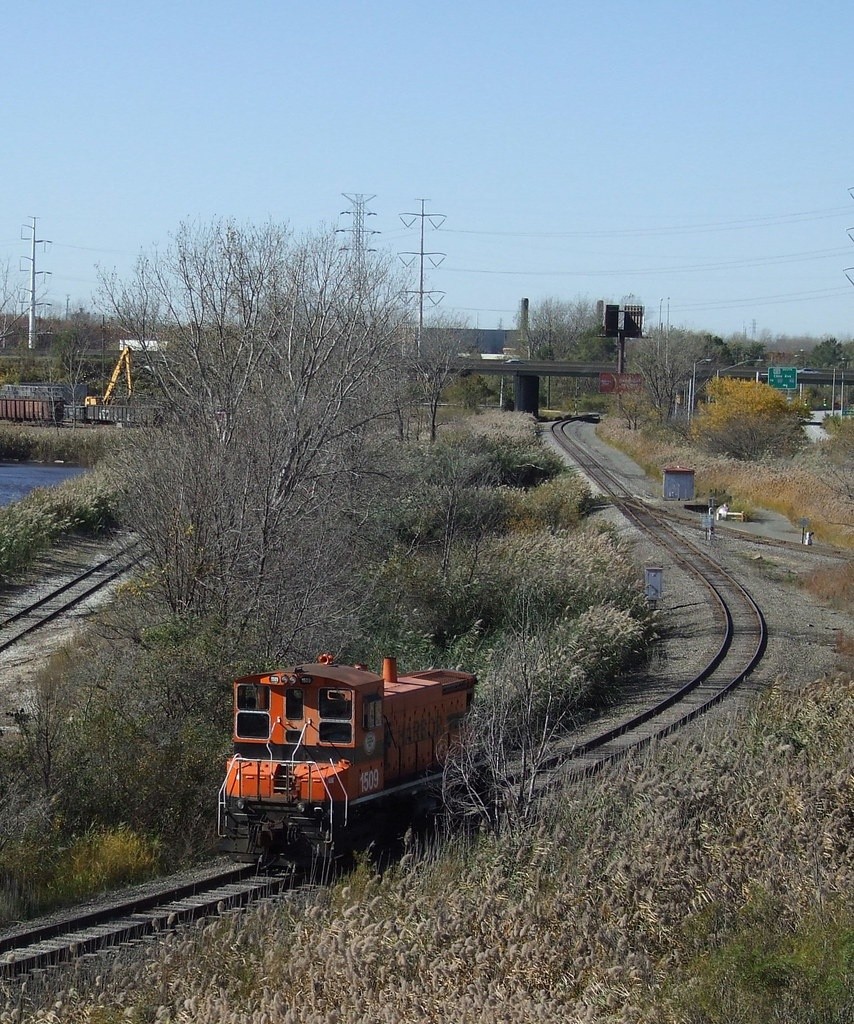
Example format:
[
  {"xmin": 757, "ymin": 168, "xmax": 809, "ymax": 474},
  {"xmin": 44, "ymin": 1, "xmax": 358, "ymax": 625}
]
[
  {"xmin": 716, "ymin": 357, "xmax": 764, "ymax": 381},
  {"xmin": 691, "ymin": 359, "xmax": 713, "ymax": 417}
]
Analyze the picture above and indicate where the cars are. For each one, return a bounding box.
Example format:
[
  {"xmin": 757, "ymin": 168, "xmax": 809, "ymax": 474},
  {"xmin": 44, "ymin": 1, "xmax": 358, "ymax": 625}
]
[{"xmin": 795, "ymin": 368, "xmax": 821, "ymax": 374}]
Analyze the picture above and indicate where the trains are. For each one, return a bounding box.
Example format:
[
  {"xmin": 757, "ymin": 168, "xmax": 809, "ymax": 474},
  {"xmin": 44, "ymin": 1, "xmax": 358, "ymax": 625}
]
[{"xmin": 224, "ymin": 651, "xmax": 501, "ymax": 872}]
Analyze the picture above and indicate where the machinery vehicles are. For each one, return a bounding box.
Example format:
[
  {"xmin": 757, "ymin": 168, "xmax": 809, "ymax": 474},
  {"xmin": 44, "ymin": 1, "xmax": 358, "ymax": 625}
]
[{"xmin": 84, "ymin": 347, "xmax": 136, "ymax": 408}]
